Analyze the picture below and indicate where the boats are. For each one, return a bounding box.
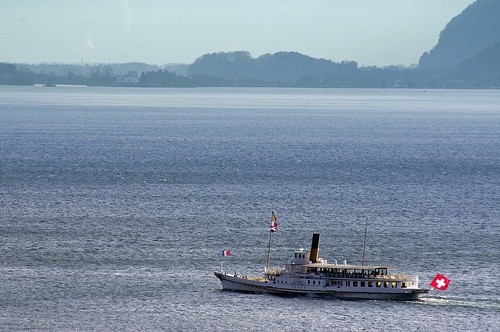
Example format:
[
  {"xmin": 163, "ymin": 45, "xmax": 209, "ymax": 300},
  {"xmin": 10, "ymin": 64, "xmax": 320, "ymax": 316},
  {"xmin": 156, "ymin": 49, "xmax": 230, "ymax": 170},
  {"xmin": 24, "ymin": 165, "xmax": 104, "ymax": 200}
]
[{"xmin": 212, "ymin": 211, "xmax": 433, "ymax": 302}]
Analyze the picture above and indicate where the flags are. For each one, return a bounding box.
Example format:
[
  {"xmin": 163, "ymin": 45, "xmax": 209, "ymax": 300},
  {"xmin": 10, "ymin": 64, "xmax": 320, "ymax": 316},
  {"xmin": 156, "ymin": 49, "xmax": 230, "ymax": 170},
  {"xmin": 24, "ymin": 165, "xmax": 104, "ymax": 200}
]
[
  {"xmin": 429, "ymin": 272, "xmax": 451, "ymax": 291},
  {"xmin": 270, "ymin": 211, "xmax": 278, "ymax": 233},
  {"xmin": 221, "ymin": 250, "xmax": 230, "ymax": 257}
]
